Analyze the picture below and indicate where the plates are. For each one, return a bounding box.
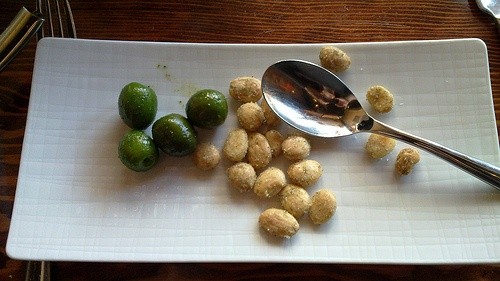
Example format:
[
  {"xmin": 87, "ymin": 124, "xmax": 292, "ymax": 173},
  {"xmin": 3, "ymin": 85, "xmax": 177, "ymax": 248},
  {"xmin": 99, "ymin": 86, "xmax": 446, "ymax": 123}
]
[{"xmin": 6, "ymin": 37, "xmax": 500, "ymax": 263}]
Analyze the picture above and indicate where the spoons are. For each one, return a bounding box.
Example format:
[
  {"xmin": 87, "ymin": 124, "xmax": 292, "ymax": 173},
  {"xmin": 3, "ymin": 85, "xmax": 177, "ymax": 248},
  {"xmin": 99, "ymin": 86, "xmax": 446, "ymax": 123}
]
[
  {"xmin": 261, "ymin": 59, "xmax": 500, "ymax": 190},
  {"xmin": 26, "ymin": 1, "xmax": 78, "ymax": 281}
]
